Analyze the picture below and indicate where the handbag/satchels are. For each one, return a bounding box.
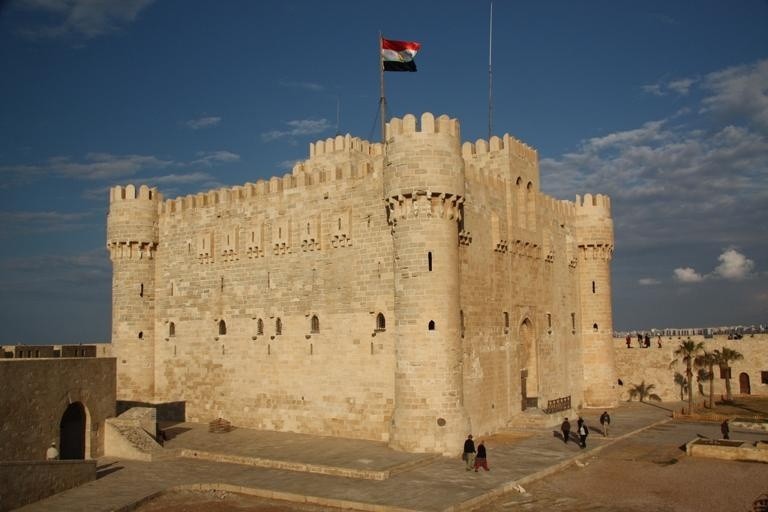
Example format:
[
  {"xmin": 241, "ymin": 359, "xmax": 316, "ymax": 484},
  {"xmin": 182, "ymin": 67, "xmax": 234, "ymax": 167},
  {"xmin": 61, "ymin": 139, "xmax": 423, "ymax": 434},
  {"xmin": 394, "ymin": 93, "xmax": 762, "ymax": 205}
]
[{"xmin": 462, "ymin": 453, "xmax": 465, "ymax": 461}]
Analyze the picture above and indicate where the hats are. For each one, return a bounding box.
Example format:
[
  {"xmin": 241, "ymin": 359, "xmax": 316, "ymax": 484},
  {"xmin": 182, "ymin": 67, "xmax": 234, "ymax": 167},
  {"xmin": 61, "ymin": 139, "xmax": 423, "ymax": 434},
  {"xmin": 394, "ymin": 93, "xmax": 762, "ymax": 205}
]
[{"xmin": 468, "ymin": 434, "xmax": 473, "ymax": 438}]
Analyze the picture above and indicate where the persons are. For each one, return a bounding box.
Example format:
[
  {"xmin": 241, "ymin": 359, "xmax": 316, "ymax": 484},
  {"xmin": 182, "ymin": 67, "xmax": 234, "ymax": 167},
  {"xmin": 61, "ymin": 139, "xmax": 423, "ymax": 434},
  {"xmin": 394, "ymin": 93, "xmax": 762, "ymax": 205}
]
[
  {"xmin": 561, "ymin": 417, "xmax": 570, "ymax": 444},
  {"xmin": 600, "ymin": 412, "xmax": 610, "ymax": 436},
  {"xmin": 474, "ymin": 440, "xmax": 490, "ymax": 472},
  {"xmin": 626, "ymin": 332, "xmax": 663, "ymax": 348},
  {"xmin": 721, "ymin": 419, "xmax": 730, "ymax": 440},
  {"xmin": 577, "ymin": 417, "xmax": 588, "ymax": 448},
  {"xmin": 463, "ymin": 435, "xmax": 476, "ymax": 471}
]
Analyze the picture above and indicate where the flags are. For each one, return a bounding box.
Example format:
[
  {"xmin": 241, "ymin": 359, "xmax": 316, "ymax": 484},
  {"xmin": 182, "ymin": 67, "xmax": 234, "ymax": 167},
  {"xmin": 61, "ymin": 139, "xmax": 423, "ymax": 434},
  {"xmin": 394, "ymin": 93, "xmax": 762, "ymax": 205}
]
[{"xmin": 382, "ymin": 36, "xmax": 421, "ymax": 71}]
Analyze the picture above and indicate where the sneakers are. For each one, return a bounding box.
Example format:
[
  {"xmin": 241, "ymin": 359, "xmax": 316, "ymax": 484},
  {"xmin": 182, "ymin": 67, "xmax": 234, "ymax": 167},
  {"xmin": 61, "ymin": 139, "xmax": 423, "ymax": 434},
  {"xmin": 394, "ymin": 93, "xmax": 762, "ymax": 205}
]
[{"xmin": 465, "ymin": 465, "xmax": 474, "ymax": 471}]
